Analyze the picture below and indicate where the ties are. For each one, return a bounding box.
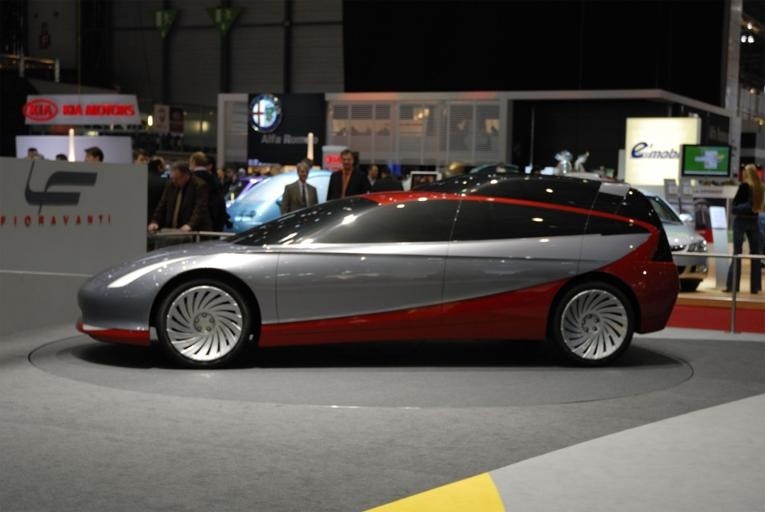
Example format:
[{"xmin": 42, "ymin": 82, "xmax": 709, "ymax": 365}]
[
  {"xmin": 301, "ymin": 186, "xmax": 306, "ymax": 207},
  {"xmin": 172, "ymin": 190, "xmax": 182, "ymax": 226}
]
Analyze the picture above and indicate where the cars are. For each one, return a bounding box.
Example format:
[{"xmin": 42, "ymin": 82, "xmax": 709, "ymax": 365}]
[
  {"xmin": 670, "ymin": 198, "xmax": 714, "ymax": 243},
  {"xmin": 636, "ymin": 187, "xmax": 710, "ymax": 291},
  {"xmin": 222, "ymin": 171, "xmax": 335, "ymax": 239},
  {"xmin": 222, "ymin": 175, "xmax": 272, "ymax": 209}
]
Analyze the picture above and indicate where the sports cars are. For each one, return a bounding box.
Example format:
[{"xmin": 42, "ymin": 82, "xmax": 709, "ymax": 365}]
[{"xmin": 72, "ymin": 171, "xmax": 681, "ymax": 367}]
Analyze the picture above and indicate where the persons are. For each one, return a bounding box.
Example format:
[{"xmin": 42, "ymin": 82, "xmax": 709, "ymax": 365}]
[
  {"xmin": 223, "ymin": 161, "xmax": 240, "ymax": 193},
  {"xmin": 56, "ymin": 153, "xmax": 67, "ymax": 160},
  {"xmin": 84, "ymin": 147, "xmax": 104, "ymax": 162},
  {"xmin": 367, "ymin": 164, "xmax": 404, "ymax": 193},
  {"xmin": 281, "ymin": 159, "xmax": 318, "ymax": 215},
  {"xmin": 326, "ymin": 149, "xmax": 370, "ymax": 202},
  {"xmin": 27, "ymin": 148, "xmax": 44, "ymax": 160},
  {"xmin": 721, "ymin": 164, "xmax": 765, "ymax": 294},
  {"xmin": 133, "ymin": 147, "xmax": 231, "ymax": 253}
]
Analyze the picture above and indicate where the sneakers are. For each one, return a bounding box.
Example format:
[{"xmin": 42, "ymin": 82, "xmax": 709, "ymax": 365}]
[{"xmin": 722, "ymin": 287, "xmax": 760, "ymax": 294}]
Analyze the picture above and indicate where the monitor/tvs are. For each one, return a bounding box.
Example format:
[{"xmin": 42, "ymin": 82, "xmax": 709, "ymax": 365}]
[{"xmin": 681, "ymin": 144, "xmax": 732, "ymax": 178}]
